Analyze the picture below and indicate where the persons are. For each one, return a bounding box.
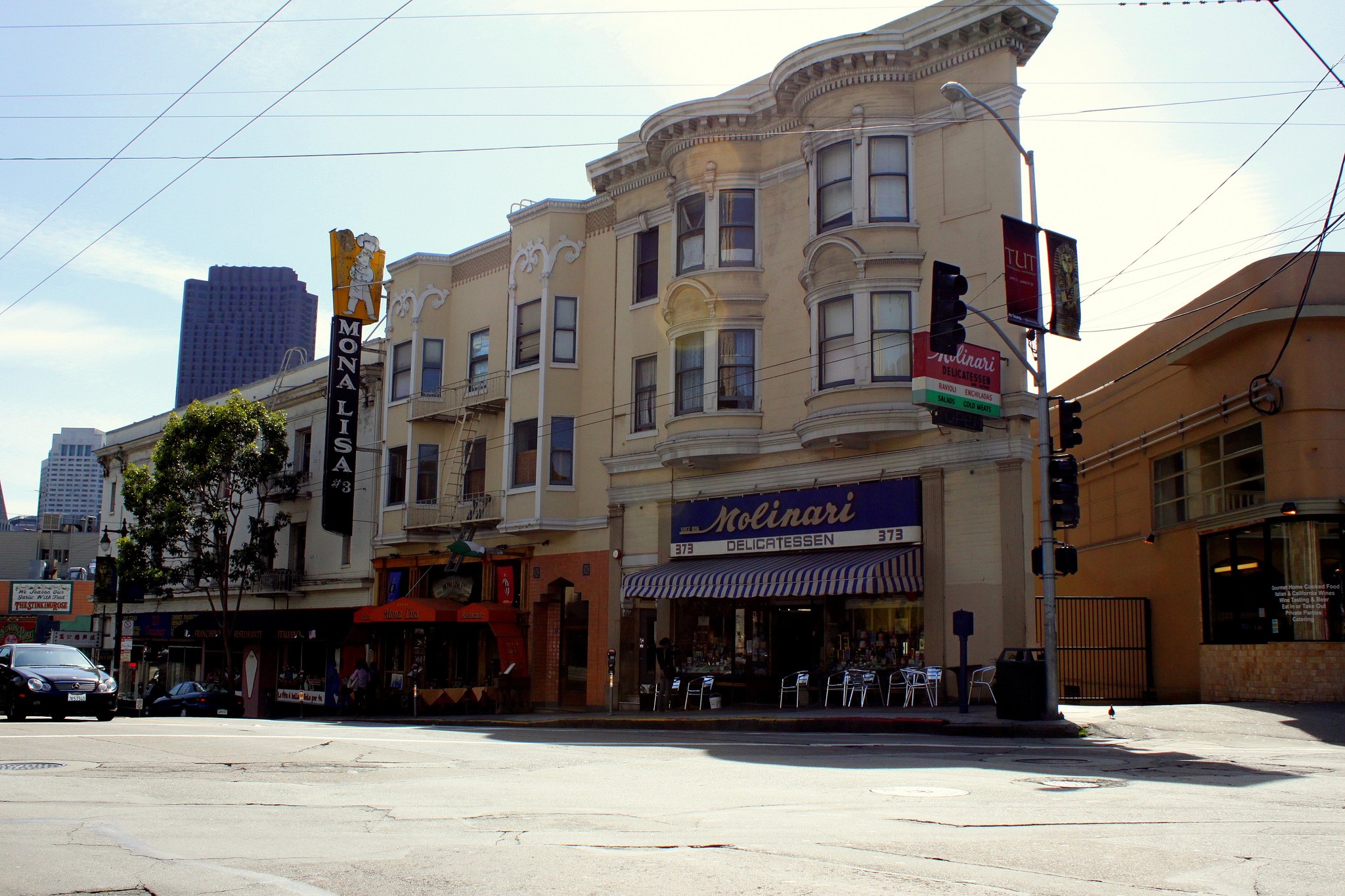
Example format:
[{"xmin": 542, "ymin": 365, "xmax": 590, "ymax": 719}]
[
  {"xmin": 656, "ymin": 637, "xmax": 675, "ymax": 713},
  {"xmin": 351, "ymin": 658, "xmax": 370, "ymax": 717}
]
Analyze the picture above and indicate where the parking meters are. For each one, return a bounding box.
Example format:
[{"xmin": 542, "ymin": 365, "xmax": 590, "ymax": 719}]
[{"xmin": 953, "ymin": 608, "xmax": 974, "ymax": 715}]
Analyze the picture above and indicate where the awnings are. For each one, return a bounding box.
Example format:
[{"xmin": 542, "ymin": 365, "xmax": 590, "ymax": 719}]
[
  {"xmin": 621, "ymin": 547, "xmax": 923, "ymax": 599},
  {"xmin": 352, "ymin": 596, "xmax": 515, "ymax": 624}
]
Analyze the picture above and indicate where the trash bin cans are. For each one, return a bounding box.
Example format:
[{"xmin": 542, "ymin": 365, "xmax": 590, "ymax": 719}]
[{"xmin": 995, "ymin": 647, "xmax": 1047, "ymax": 722}]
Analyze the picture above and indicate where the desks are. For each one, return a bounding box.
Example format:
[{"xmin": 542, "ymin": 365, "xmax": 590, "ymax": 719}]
[
  {"xmin": 938, "ymin": 665, "xmax": 982, "ymax": 705},
  {"xmin": 415, "ymin": 686, "xmax": 502, "ymax": 716}
]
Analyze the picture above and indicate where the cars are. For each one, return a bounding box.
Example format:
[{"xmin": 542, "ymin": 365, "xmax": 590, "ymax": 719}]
[
  {"xmin": 147, "ymin": 681, "xmax": 245, "ymax": 719},
  {"xmin": 0, "ymin": 643, "xmax": 120, "ymax": 722}
]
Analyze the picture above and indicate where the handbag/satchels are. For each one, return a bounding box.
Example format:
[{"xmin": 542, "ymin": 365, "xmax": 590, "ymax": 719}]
[{"xmin": 663, "ymin": 665, "xmax": 676, "ymax": 679}]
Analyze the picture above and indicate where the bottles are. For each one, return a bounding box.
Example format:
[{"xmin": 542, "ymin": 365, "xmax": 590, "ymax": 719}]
[
  {"xmin": 727, "ymin": 652, "xmax": 731, "ymax": 663},
  {"xmin": 720, "ymin": 657, "xmax": 724, "ymax": 664}
]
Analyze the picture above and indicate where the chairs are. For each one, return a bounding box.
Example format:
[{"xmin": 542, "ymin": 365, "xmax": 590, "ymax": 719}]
[
  {"xmin": 779, "ymin": 670, "xmax": 823, "ymax": 710},
  {"xmin": 684, "ymin": 675, "xmax": 715, "ymax": 710},
  {"xmin": 825, "ymin": 666, "xmax": 998, "ymax": 708},
  {"xmin": 653, "ymin": 677, "xmax": 683, "ymax": 711}
]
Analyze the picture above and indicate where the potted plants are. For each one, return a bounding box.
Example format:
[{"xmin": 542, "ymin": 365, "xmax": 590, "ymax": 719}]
[{"xmin": 634, "ymin": 675, "xmax": 658, "ymax": 712}]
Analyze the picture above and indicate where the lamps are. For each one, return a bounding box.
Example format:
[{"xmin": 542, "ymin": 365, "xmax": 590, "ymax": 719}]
[
  {"xmin": 541, "ymin": 539, "xmax": 548, "ymax": 547},
  {"xmin": 1281, "ymin": 501, "xmax": 1297, "ymax": 514},
  {"xmin": 495, "ymin": 544, "xmax": 507, "ymax": 550},
  {"xmin": 1143, "ymin": 533, "xmax": 1157, "ymax": 546},
  {"xmin": 428, "ymin": 550, "xmax": 440, "ymax": 555},
  {"xmin": 389, "ymin": 553, "xmax": 400, "ymax": 560}
]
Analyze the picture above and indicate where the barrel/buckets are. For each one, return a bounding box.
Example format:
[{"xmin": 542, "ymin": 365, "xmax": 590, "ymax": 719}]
[{"xmin": 709, "ymin": 693, "xmax": 722, "ymax": 710}]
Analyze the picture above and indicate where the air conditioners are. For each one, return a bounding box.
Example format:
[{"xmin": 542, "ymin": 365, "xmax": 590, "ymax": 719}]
[{"xmin": 81, "ymin": 515, "xmax": 97, "ymax": 533}]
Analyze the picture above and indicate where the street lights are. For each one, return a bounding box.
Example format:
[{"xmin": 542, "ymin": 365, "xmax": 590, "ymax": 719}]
[
  {"xmin": 99, "ymin": 517, "xmax": 130, "ymax": 682},
  {"xmin": 939, "ymin": 80, "xmax": 1059, "ymax": 719}
]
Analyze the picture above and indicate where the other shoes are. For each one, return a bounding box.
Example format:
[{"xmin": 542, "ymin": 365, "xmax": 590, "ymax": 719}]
[
  {"xmin": 665, "ymin": 709, "xmax": 673, "ymax": 712},
  {"xmin": 657, "ymin": 710, "xmax": 663, "ymax": 712}
]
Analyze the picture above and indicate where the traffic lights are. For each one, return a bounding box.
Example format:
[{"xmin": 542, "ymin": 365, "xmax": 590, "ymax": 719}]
[
  {"xmin": 1057, "ymin": 397, "xmax": 1083, "ymax": 449},
  {"xmin": 930, "ymin": 260, "xmax": 968, "ymax": 356},
  {"xmin": 1048, "ymin": 454, "xmax": 1080, "ymax": 525},
  {"xmin": 1054, "ymin": 546, "xmax": 1078, "ymax": 575}
]
